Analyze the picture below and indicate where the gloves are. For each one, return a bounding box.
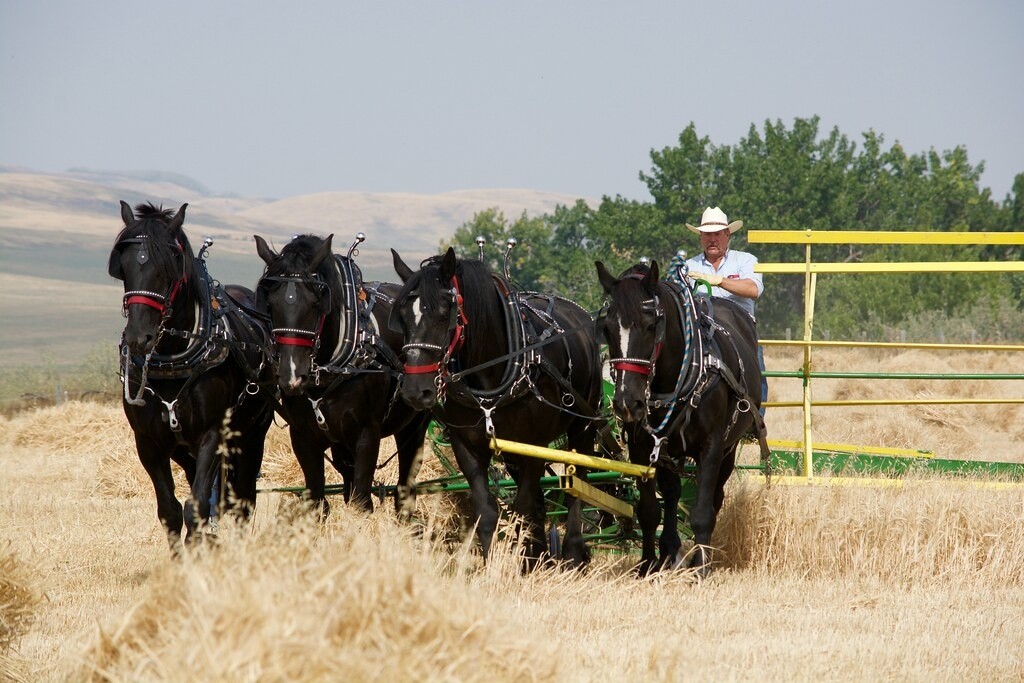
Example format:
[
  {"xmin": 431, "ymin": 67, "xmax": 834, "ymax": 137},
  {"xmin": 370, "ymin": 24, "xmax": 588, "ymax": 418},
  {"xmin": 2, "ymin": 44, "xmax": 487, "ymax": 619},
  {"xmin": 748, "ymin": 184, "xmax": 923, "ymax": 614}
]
[{"xmin": 689, "ymin": 271, "xmax": 723, "ymax": 288}]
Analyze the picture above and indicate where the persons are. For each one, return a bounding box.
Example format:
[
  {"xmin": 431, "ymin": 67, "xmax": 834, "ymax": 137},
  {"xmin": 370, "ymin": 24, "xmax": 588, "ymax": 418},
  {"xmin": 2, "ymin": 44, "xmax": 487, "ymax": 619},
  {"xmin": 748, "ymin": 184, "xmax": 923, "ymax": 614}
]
[{"xmin": 679, "ymin": 206, "xmax": 764, "ymax": 322}]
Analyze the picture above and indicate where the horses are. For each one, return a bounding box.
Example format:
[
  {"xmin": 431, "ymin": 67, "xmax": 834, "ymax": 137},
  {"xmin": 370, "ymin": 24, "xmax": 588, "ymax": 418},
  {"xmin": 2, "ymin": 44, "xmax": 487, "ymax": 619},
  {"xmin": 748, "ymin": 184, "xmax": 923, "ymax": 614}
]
[{"xmin": 108, "ymin": 200, "xmax": 764, "ymax": 580}]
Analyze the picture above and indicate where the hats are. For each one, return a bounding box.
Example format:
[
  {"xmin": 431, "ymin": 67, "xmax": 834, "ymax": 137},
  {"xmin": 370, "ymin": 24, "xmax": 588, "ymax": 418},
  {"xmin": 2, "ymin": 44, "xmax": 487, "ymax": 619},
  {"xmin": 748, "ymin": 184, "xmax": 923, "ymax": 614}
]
[{"xmin": 685, "ymin": 206, "xmax": 743, "ymax": 235}]
